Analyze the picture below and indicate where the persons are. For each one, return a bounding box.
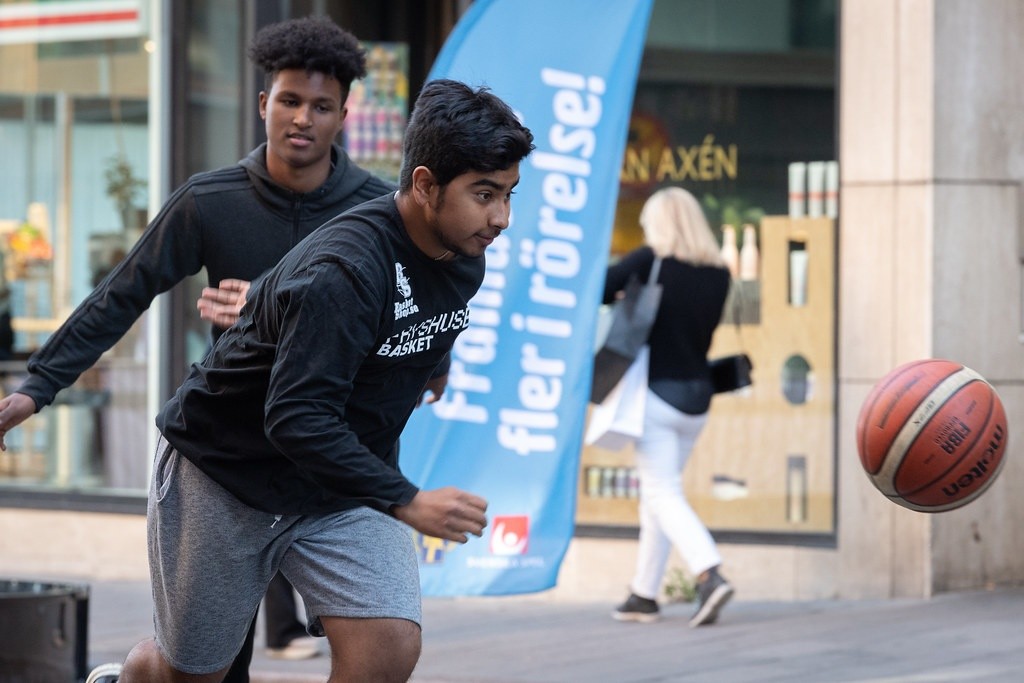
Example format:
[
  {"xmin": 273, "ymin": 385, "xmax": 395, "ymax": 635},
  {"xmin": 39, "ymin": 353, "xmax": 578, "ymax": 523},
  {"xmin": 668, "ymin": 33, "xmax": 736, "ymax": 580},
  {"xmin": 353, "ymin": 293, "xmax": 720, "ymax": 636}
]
[
  {"xmin": 90, "ymin": 77, "xmax": 534, "ymax": 683},
  {"xmin": 261, "ymin": 559, "xmax": 321, "ymax": 662},
  {"xmin": 0, "ymin": 18, "xmax": 394, "ymax": 683},
  {"xmin": 601, "ymin": 187, "xmax": 734, "ymax": 627}
]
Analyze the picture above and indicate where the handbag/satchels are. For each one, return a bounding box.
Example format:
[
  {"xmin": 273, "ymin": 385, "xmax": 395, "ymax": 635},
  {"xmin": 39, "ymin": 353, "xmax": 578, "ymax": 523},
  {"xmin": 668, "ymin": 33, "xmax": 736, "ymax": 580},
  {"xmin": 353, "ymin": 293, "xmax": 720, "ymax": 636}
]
[
  {"xmin": 709, "ymin": 354, "xmax": 752, "ymax": 393},
  {"xmin": 589, "ymin": 256, "xmax": 663, "ymax": 404}
]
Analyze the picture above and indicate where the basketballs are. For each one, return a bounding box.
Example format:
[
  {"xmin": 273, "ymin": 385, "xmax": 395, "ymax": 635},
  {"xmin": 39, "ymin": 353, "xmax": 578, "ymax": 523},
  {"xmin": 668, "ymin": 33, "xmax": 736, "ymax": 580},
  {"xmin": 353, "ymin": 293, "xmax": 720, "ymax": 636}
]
[{"xmin": 855, "ymin": 359, "xmax": 1009, "ymax": 514}]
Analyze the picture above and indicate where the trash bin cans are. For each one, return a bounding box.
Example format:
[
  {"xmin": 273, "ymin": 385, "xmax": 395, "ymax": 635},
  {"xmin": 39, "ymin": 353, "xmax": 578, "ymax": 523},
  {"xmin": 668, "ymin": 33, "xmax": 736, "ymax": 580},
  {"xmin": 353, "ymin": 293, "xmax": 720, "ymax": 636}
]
[{"xmin": 0, "ymin": 578, "xmax": 90, "ymax": 683}]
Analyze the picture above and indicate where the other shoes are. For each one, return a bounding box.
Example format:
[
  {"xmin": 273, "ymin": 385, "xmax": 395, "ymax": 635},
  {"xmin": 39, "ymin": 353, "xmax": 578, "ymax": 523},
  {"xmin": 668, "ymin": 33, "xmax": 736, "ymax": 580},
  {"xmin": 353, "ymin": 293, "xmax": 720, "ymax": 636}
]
[
  {"xmin": 611, "ymin": 595, "xmax": 659, "ymax": 622},
  {"xmin": 689, "ymin": 572, "xmax": 734, "ymax": 625},
  {"xmin": 85, "ymin": 663, "xmax": 123, "ymax": 683}
]
